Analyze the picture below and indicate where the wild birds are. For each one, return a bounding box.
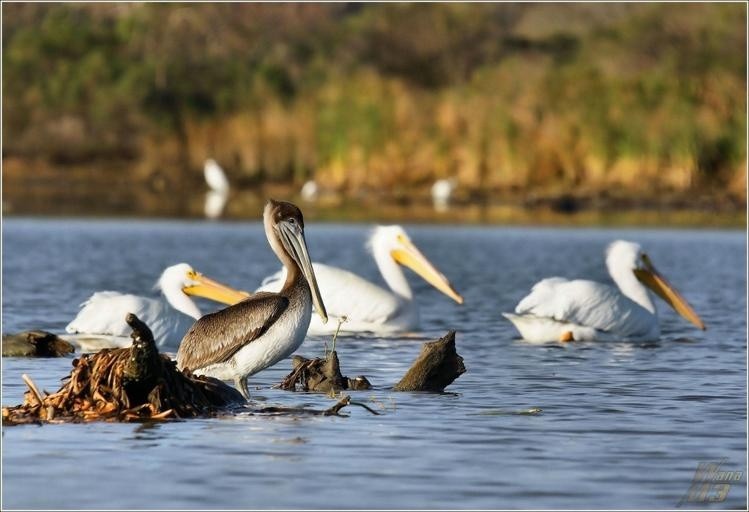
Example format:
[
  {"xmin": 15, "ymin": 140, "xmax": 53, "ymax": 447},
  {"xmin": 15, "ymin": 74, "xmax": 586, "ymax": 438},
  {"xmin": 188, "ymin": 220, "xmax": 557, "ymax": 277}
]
[
  {"xmin": 174, "ymin": 199, "xmax": 329, "ymax": 400},
  {"xmin": 250, "ymin": 222, "xmax": 464, "ymax": 338},
  {"xmin": 501, "ymin": 238, "xmax": 707, "ymax": 346},
  {"xmin": 63, "ymin": 261, "xmax": 249, "ymax": 351}
]
